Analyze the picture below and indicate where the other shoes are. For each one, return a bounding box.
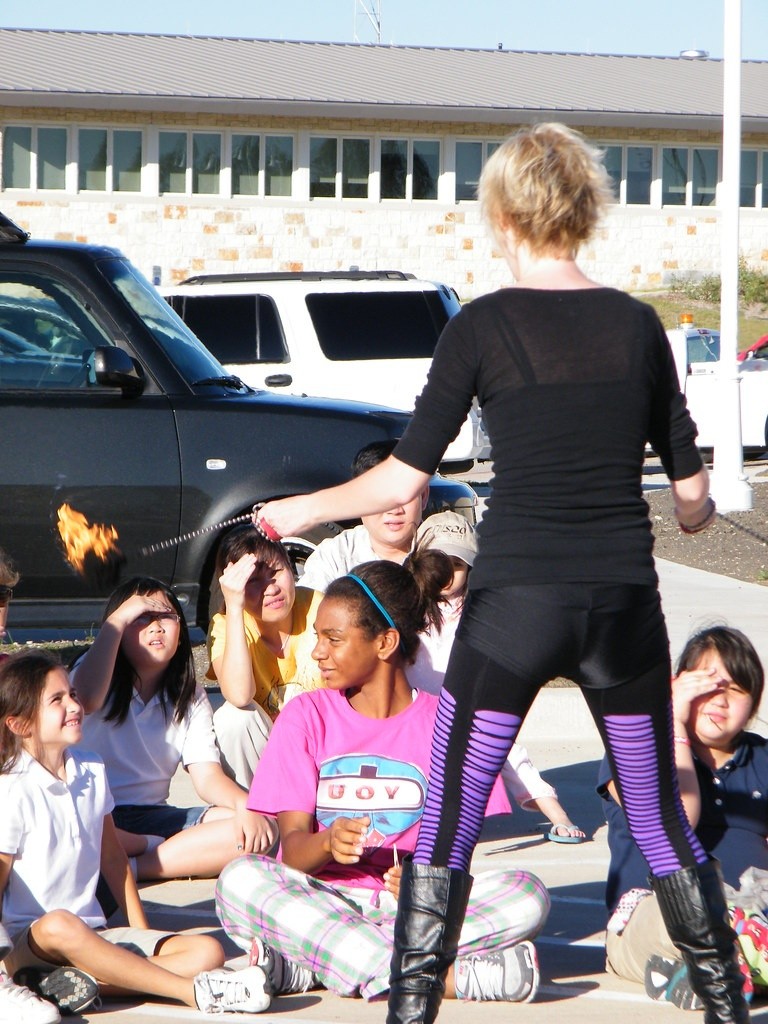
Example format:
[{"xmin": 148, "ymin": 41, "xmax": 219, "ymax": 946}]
[{"xmin": 644, "ymin": 956, "xmax": 705, "ymax": 1010}]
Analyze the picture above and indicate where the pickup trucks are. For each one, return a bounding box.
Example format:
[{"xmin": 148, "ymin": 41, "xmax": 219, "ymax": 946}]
[{"xmin": 641, "ymin": 314, "xmax": 768, "ymax": 463}]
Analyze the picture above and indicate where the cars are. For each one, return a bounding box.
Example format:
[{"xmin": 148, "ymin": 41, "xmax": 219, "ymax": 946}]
[{"xmin": 737, "ymin": 333, "xmax": 768, "ymax": 363}]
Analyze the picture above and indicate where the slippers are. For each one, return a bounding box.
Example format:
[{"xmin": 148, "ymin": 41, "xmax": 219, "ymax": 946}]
[{"xmin": 544, "ymin": 824, "xmax": 582, "ymax": 843}]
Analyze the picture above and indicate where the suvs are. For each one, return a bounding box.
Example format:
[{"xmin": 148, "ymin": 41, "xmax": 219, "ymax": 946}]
[
  {"xmin": 150, "ymin": 270, "xmax": 494, "ymax": 476},
  {"xmin": 0, "ymin": 209, "xmax": 479, "ymax": 666}
]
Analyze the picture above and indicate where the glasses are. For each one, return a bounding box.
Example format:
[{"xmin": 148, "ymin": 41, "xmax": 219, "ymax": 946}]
[
  {"xmin": 0, "ymin": 586, "xmax": 12, "ymax": 608},
  {"xmin": 131, "ymin": 613, "xmax": 182, "ymax": 628}
]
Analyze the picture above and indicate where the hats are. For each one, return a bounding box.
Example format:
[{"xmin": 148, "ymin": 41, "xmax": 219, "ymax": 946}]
[{"xmin": 411, "ymin": 510, "xmax": 478, "ymax": 567}]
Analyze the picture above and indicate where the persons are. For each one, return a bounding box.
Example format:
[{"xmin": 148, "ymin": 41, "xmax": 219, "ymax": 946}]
[
  {"xmin": 0, "ymin": 652, "xmax": 275, "ymax": 1024},
  {"xmin": 593, "ymin": 624, "xmax": 768, "ymax": 1011},
  {"xmin": 204, "ymin": 438, "xmax": 585, "ymax": 844},
  {"xmin": 250, "ymin": 124, "xmax": 750, "ymax": 1024},
  {"xmin": 216, "ymin": 548, "xmax": 548, "ymax": 1004},
  {"xmin": 69, "ymin": 574, "xmax": 285, "ymax": 880},
  {"xmin": 48, "ymin": 325, "xmax": 68, "ymax": 351}
]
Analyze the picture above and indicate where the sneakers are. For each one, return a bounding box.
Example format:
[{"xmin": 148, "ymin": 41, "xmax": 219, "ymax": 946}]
[
  {"xmin": 454, "ymin": 940, "xmax": 540, "ymax": 1003},
  {"xmin": 15, "ymin": 966, "xmax": 101, "ymax": 1015},
  {"xmin": 0, "ymin": 972, "xmax": 60, "ymax": 1024},
  {"xmin": 249, "ymin": 937, "xmax": 316, "ymax": 995},
  {"xmin": 193, "ymin": 967, "xmax": 272, "ymax": 1013}
]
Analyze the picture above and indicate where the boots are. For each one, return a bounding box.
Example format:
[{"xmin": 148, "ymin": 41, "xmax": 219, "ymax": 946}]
[
  {"xmin": 651, "ymin": 854, "xmax": 749, "ymax": 1024},
  {"xmin": 386, "ymin": 854, "xmax": 472, "ymax": 1024}
]
